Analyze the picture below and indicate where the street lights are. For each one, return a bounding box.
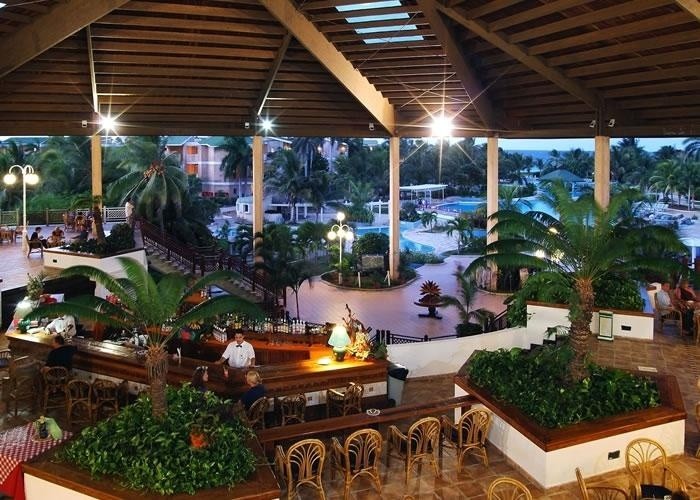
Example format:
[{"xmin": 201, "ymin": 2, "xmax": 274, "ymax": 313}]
[
  {"xmin": 328, "ymin": 212, "xmax": 354, "ymax": 285},
  {"xmin": 3, "ymin": 165, "xmax": 40, "ymax": 252}
]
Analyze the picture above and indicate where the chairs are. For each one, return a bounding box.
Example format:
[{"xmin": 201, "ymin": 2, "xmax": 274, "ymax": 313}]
[
  {"xmin": 655, "ymin": 291, "xmax": 684, "ymax": 339},
  {"xmin": 331, "ymin": 428, "xmax": 386, "ymax": 499},
  {"xmin": 327, "ymin": 382, "xmax": 363, "ymax": 416},
  {"xmin": 277, "ymin": 393, "xmax": 304, "ymax": 424},
  {"xmin": 0, "ymin": 350, "xmax": 268, "ymax": 431},
  {"xmin": 488, "ymin": 475, "xmax": 531, "ymax": 500},
  {"xmin": 627, "ymin": 435, "xmax": 687, "ymax": 499},
  {"xmin": 390, "ymin": 417, "xmax": 441, "ymax": 483},
  {"xmin": 442, "ymin": 407, "xmax": 492, "ymax": 474},
  {"xmin": 0, "ymin": 204, "xmax": 98, "ymax": 258},
  {"xmin": 575, "ymin": 466, "xmax": 627, "ymax": 499},
  {"xmin": 274, "ymin": 438, "xmax": 327, "ymax": 500}
]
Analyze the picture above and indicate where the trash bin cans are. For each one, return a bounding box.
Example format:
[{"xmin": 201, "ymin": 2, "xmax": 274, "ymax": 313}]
[{"xmin": 386, "ymin": 364, "xmax": 410, "ymax": 407}]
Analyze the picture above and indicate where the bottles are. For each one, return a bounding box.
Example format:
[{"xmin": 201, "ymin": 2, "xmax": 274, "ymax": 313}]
[
  {"xmin": 224, "ymin": 311, "xmax": 308, "ymax": 335},
  {"xmin": 159, "ymin": 318, "xmax": 177, "ymax": 331},
  {"xmin": 212, "ymin": 326, "xmax": 229, "ymax": 344}
]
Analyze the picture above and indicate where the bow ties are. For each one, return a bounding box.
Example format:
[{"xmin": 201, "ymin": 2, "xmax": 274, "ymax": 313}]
[
  {"xmin": 60, "ymin": 318, "xmax": 64, "ymax": 320},
  {"xmin": 236, "ymin": 345, "xmax": 242, "ymax": 347}
]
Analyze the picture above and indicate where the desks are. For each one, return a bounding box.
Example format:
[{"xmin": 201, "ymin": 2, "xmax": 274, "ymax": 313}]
[{"xmin": 686, "ymin": 299, "xmax": 700, "ymax": 320}]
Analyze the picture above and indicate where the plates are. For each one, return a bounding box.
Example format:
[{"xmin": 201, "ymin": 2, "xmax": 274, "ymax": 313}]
[{"xmin": 32, "ymin": 434, "xmax": 58, "ymax": 442}]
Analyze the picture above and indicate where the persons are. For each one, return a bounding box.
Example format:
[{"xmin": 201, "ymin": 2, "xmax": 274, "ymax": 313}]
[
  {"xmin": 189, "ymin": 365, "xmax": 209, "ymax": 392},
  {"xmin": 240, "ymin": 370, "xmax": 267, "ymax": 429},
  {"xmin": 8, "ymin": 202, "xmax": 110, "ymax": 396},
  {"xmin": 674, "ymin": 277, "xmax": 697, "ymax": 332},
  {"xmin": 657, "ymin": 280, "xmax": 695, "ymax": 339},
  {"xmin": 214, "ymin": 328, "xmax": 256, "ymax": 369}
]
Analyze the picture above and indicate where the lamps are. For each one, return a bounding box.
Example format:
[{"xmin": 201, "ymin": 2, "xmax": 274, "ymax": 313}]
[{"xmin": 328, "ymin": 324, "xmax": 351, "ymax": 362}]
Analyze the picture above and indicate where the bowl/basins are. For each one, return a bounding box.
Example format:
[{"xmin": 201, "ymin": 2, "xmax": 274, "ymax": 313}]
[{"xmin": 367, "ymin": 409, "xmax": 380, "ymax": 417}]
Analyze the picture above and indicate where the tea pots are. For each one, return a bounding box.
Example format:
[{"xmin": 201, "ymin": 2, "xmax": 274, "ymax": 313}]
[{"xmin": 35, "ymin": 417, "xmax": 49, "ymax": 439}]
